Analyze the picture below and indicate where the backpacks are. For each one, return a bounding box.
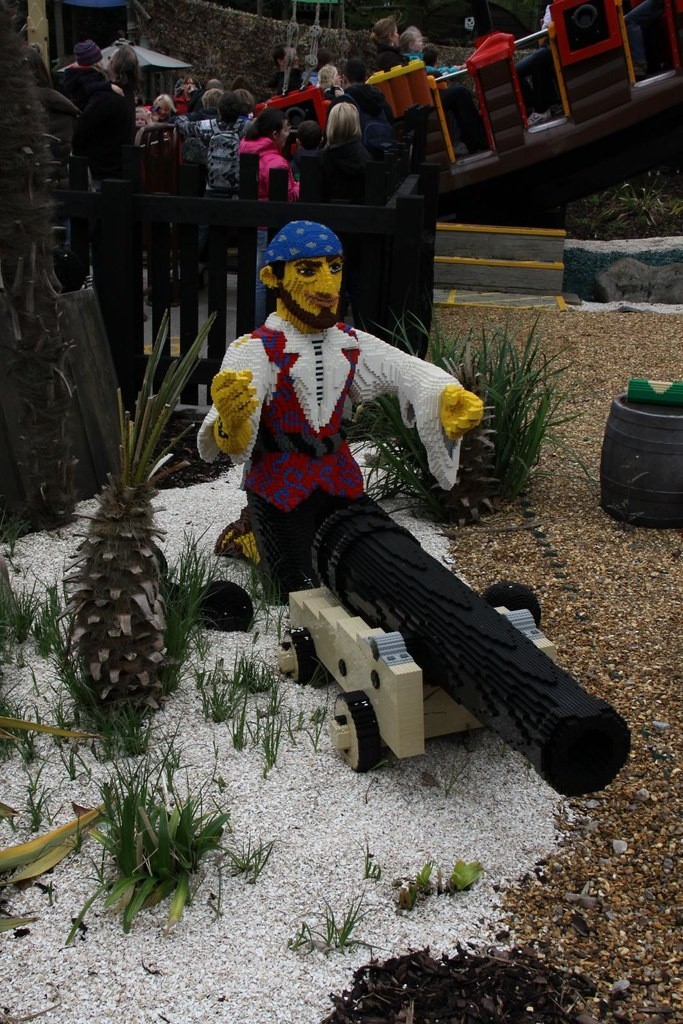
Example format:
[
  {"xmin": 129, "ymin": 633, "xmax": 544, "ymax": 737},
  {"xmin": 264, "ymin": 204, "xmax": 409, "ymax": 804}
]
[{"xmin": 206, "ymin": 119, "xmax": 249, "ymax": 191}]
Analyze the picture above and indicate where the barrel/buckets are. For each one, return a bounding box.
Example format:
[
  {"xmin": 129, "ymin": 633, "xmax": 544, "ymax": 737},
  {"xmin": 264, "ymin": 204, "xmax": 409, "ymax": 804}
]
[{"xmin": 600, "ymin": 392, "xmax": 683, "ymax": 529}]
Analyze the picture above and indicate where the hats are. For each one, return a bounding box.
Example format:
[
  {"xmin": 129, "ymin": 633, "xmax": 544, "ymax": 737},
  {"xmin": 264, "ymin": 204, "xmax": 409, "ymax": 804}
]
[{"xmin": 73, "ymin": 40, "xmax": 102, "ymax": 66}]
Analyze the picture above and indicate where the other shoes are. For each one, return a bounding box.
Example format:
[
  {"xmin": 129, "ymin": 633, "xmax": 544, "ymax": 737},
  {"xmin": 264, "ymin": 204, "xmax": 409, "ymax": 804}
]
[{"xmin": 528, "ymin": 109, "xmax": 552, "ymax": 124}]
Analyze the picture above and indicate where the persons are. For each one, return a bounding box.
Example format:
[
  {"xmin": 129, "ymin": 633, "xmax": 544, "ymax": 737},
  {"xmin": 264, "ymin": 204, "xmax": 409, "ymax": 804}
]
[
  {"xmin": 25, "ymin": 18, "xmax": 558, "ymax": 324},
  {"xmin": 197, "ymin": 219, "xmax": 486, "ymax": 606}
]
